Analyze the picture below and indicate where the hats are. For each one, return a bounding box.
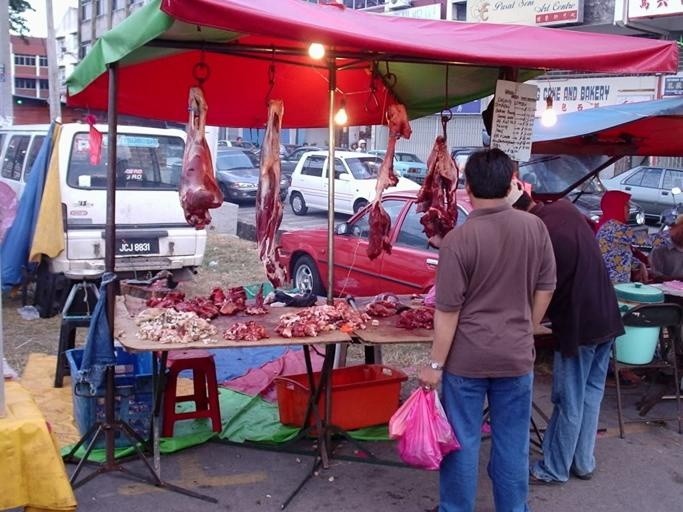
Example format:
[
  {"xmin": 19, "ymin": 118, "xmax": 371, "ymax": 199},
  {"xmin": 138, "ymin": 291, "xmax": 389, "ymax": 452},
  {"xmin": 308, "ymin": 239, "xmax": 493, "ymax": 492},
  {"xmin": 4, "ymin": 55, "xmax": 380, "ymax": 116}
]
[{"xmin": 506, "ymin": 179, "xmax": 525, "ymax": 205}]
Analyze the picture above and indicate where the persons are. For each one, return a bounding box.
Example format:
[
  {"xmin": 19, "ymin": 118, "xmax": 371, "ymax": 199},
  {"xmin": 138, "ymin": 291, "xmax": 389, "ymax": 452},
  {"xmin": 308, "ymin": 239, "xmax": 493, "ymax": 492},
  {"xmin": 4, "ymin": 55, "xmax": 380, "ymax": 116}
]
[
  {"xmin": 303, "ymin": 142, "xmax": 308, "ymax": 147},
  {"xmin": 595, "ymin": 191, "xmax": 648, "ymax": 389},
  {"xmin": 417, "ymin": 149, "xmax": 557, "ymax": 512},
  {"xmin": 351, "ymin": 142, "xmax": 358, "ymax": 152},
  {"xmin": 356, "ymin": 139, "xmax": 367, "ymax": 154},
  {"xmin": 648, "ymin": 214, "xmax": 683, "ymax": 393},
  {"xmin": 232, "ymin": 137, "xmax": 242, "ymax": 147},
  {"xmin": 505, "ymin": 183, "xmax": 626, "ymax": 485}
]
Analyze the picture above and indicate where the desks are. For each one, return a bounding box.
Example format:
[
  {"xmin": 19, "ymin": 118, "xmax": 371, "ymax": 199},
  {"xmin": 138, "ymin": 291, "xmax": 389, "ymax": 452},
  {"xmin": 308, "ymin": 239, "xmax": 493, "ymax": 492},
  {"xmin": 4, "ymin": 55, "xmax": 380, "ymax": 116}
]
[
  {"xmin": 0, "ymin": 354, "xmax": 46, "ymax": 512},
  {"xmin": 113, "ymin": 302, "xmax": 353, "ymax": 486},
  {"xmin": 649, "ymin": 281, "xmax": 683, "ymax": 302},
  {"xmin": 315, "ymin": 292, "xmax": 554, "ymax": 449}
]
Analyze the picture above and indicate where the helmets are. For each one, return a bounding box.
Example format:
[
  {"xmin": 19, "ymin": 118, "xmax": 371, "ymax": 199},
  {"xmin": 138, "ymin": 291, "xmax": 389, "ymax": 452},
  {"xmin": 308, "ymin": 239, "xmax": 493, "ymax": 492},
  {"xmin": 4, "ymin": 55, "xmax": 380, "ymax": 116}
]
[{"xmin": 358, "ymin": 139, "xmax": 366, "ymax": 148}]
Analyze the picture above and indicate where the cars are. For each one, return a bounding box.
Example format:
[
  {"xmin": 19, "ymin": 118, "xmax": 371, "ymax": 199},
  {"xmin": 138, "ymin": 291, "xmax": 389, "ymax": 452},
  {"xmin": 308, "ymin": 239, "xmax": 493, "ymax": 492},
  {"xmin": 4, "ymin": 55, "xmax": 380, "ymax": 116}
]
[
  {"xmin": 216, "ymin": 146, "xmax": 288, "ymax": 204},
  {"xmin": 279, "ymin": 192, "xmax": 526, "ymax": 297},
  {"xmin": 599, "ymin": 165, "xmax": 682, "ymax": 217},
  {"xmin": 285, "ymin": 150, "xmax": 423, "ymax": 216},
  {"xmin": 451, "ymin": 149, "xmax": 645, "ymax": 236},
  {"xmin": 214, "ymin": 140, "xmax": 427, "ymax": 178}
]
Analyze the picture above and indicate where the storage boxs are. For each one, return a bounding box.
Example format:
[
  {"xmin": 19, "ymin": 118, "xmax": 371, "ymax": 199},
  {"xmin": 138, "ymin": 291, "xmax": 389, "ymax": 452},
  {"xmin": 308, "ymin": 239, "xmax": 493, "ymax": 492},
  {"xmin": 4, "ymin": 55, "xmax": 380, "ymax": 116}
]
[
  {"xmin": 273, "ymin": 363, "xmax": 408, "ymax": 433},
  {"xmin": 65, "ymin": 348, "xmax": 170, "ymax": 450}
]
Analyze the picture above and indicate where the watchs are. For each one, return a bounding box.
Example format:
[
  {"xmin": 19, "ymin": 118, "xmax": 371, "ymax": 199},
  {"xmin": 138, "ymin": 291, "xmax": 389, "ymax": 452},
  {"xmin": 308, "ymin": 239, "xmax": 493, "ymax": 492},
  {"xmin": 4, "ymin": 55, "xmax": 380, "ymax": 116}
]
[{"xmin": 428, "ymin": 360, "xmax": 444, "ymax": 371}]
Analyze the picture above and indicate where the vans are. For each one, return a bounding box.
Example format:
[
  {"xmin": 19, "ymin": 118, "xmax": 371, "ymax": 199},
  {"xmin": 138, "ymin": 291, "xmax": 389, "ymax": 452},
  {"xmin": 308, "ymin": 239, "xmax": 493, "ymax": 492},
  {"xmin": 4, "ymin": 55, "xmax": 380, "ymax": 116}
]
[{"xmin": 0, "ymin": 122, "xmax": 207, "ymax": 281}]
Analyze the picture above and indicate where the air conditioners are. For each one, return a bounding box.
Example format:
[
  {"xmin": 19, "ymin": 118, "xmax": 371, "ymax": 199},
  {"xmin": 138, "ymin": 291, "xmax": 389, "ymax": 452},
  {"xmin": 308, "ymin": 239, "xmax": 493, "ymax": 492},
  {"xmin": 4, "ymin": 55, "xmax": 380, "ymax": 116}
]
[{"xmin": 389, "ymin": 0, "xmax": 411, "ymax": 10}]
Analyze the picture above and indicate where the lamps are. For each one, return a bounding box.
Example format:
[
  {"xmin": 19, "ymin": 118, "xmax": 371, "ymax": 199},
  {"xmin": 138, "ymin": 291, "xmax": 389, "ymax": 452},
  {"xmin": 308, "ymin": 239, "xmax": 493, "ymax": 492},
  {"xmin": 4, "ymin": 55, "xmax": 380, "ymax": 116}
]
[
  {"xmin": 307, "ymin": 41, "xmax": 325, "ymax": 60},
  {"xmin": 334, "ymin": 96, "xmax": 348, "ymax": 124},
  {"xmin": 540, "ymin": 95, "xmax": 559, "ymax": 127}
]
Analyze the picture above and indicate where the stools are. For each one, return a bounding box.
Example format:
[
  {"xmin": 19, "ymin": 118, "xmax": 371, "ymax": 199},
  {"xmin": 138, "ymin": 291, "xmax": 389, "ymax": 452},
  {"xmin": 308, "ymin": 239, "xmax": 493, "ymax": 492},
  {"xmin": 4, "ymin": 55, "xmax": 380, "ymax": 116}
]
[
  {"xmin": 161, "ymin": 349, "xmax": 222, "ymax": 438},
  {"xmin": 53, "ymin": 316, "xmax": 91, "ymax": 387}
]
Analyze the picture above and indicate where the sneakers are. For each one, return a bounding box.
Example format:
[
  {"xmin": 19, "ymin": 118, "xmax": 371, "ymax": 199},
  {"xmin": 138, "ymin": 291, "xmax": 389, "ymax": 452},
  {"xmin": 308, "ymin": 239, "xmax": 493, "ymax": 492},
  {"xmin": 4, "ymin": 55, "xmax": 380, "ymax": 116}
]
[
  {"xmin": 572, "ymin": 465, "xmax": 594, "ymax": 480},
  {"xmin": 528, "ymin": 464, "xmax": 565, "ymax": 486}
]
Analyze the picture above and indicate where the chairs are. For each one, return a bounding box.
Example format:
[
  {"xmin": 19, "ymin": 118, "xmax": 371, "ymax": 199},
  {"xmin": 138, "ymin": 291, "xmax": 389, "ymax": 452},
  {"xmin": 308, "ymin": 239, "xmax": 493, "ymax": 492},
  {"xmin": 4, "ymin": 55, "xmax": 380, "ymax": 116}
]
[
  {"xmin": 612, "ymin": 303, "xmax": 683, "ymax": 438},
  {"xmin": 523, "ymin": 174, "xmax": 536, "ymax": 191}
]
